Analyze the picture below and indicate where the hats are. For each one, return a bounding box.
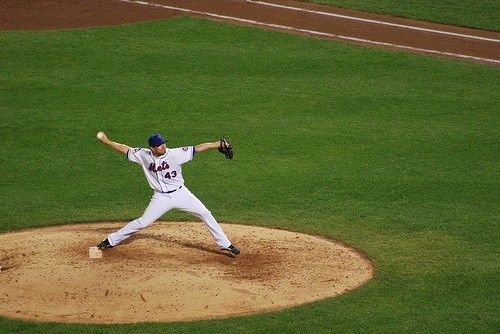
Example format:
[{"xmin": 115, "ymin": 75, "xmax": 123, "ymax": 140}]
[{"xmin": 148, "ymin": 134, "xmax": 168, "ymax": 147}]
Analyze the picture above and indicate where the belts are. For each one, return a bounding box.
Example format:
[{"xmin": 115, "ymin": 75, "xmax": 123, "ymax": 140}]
[{"xmin": 163, "ymin": 186, "xmax": 182, "ymax": 193}]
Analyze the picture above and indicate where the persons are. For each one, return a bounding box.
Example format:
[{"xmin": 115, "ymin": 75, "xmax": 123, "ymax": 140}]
[{"xmin": 96, "ymin": 131, "xmax": 241, "ymax": 257}]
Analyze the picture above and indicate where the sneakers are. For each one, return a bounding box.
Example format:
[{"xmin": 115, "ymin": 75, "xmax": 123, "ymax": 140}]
[
  {"xmin": 97, "ymin": 239, "xmax": 114, "ymax": 250},
  {"xmin": 227, "ymin": 245, "xmax": 240, "ymax": 254}
]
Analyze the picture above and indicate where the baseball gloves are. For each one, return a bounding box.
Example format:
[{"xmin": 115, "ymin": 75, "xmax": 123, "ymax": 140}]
[{"xmin": 218, "ymin": 138, "xmax": 233, "ymax": 160}]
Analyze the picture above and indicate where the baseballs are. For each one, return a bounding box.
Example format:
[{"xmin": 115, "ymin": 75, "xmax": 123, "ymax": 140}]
[{"xmin": 97, "ymin": 132, "xmax": 103, "ymax": 139}]
